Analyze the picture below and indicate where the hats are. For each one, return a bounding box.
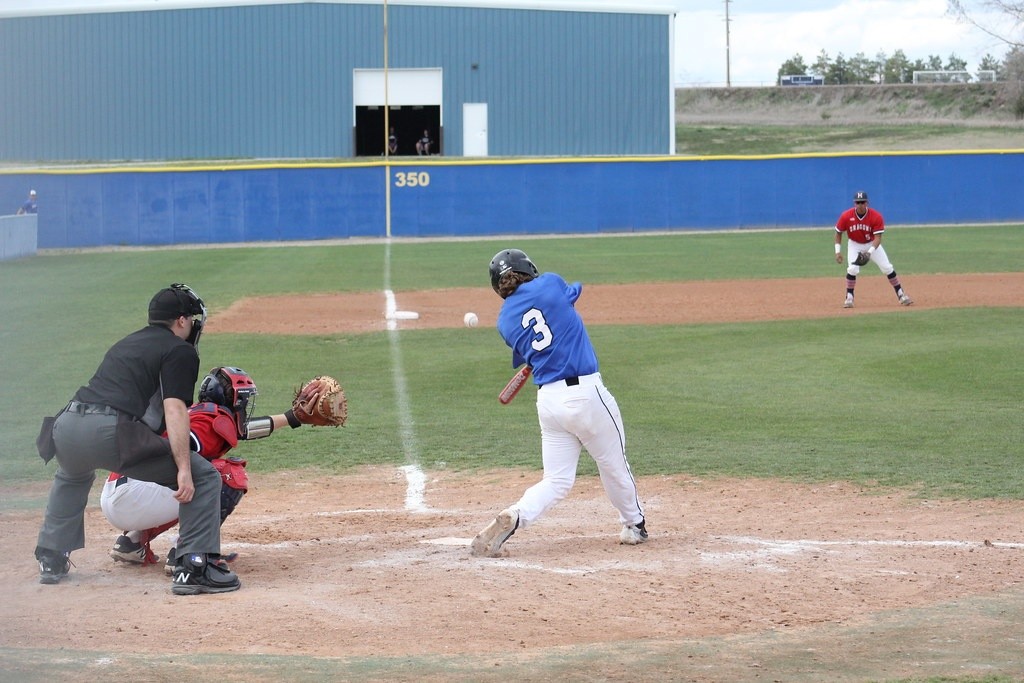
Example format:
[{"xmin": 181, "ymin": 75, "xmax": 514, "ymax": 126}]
[
  {"xmin": 853, "ymin": 191, "xmax": 868, "ymax": 201},
  {"xmin": 148, "ymin": 287, "xmax": 194, "ymax": 326}
]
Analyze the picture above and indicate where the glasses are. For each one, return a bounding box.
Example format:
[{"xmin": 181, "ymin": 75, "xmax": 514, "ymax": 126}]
[{"xmin": 856, "ymin": 202, "xmax": 865, "ymax": 204}]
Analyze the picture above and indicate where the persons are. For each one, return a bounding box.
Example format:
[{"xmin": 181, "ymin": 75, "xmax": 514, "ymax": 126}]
[
  {"xmin": 101, "ymin": 367, "xmax": 319, "ymax": 576},
  {"xmin": 388, "ymin": 127, "xmax": 399, "ymax": 156},
  {"xmin": 416, "ymin": 129, "xmax": 433, "ymax": 155},
  {"xmin": 834, "ymin": 189, "xmax": 914, "ymax": 308},
  {"xmin": 899, "ymin": 66, "xmax": 905, "ymax": 83},
  {"xmin": 470, "ymin": 249, "xmax": 649, "ymax": 557},
  {"xmin": 881, "ymin": 72, "xmax": 885, "ymax": 84},
  {"xmin": 34, "ymin": 283, "xmax": 241, "ymax": 595}
]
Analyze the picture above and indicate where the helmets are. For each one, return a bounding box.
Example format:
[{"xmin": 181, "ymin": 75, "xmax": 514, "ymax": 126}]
[
  {"xmin": 198, "ymin": 367, "xmax": 259, "ymax": 429},
  {"xmin": 489, "ymin": 248, "xmax": 540, "ymax": 299}
]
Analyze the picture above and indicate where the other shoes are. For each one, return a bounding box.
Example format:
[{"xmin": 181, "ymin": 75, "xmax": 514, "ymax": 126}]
[
  {"xmin": 843, "ymin": 301, "xmax": 856, "ymax": 308},
  {"xmin": 899, "ymin": 295, "xmax": 913, "ymax": 306}
]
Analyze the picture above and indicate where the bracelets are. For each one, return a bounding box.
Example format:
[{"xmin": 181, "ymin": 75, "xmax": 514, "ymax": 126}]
[
  {"xmin": 868, "ymin": 246, "xmax": 875, "ymax": 254},
  {"xmin": 835, "ymin": 243, "xmax": 841, "ymax": 254}
]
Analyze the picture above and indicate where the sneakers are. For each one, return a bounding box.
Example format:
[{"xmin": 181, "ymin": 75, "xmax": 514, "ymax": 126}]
[
  {"xmin": 165, "ymin": 548, "xmax": 239, "ymax": 575},
  {"xmin": 170, "ymin": 563, "xmax": 241, "ymax": 595},
  {"xmin": 619, "ymin": 524, "xmax": 649, "ymax": 545},
  {"xmin": 37, "ymin": 554, "xmax": 72, "ymax": 584},
  {"xmin": 110, "ymin": 535, "xmax": 159, "ymax": 563},
  {"xmin": 469, "ymin": 509, "xmax": 518, "ymax": 554}
]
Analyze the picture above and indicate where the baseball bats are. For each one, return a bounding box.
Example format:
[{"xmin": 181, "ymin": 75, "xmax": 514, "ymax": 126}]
[{"xmin": 499, "ymin": 363, "xmax": 534, "ymax": 404}]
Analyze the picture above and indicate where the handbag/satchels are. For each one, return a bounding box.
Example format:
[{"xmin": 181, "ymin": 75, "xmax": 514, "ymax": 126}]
[
  {"xmin": 36, "ymin": 404, "xmax": 68, "ymax": 466},
  {"xmin": 114, "ymin": 411, "xmax": 170, "ymax": 469}
]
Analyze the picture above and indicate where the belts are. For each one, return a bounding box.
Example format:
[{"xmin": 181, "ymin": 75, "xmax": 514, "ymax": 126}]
[
  {"xmin": 115, "ymin": 477, "xmax": 127, "ymax": 486},
  {"xmin": 538, "ymin": 377, "xmax": 579, "ymax": 388},
  {"xmin": 64, "ymin": 402, "xmax": 116, "ymax": 417}
]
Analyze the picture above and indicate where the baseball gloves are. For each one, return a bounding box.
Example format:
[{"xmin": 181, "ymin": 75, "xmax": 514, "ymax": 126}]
[
  {"xmin": 852, "ymin": 252, "xmax": 871, "ymax": 266},
  {"xmin": 290, "ymin": 375, "xmax": 348, "ymax": 429}
]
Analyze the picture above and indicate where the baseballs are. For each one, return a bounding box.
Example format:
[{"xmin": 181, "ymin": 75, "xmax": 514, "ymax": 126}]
[{"xmin": 464, "ymin": 312, "xmax": 480, "ymax": 328}]
